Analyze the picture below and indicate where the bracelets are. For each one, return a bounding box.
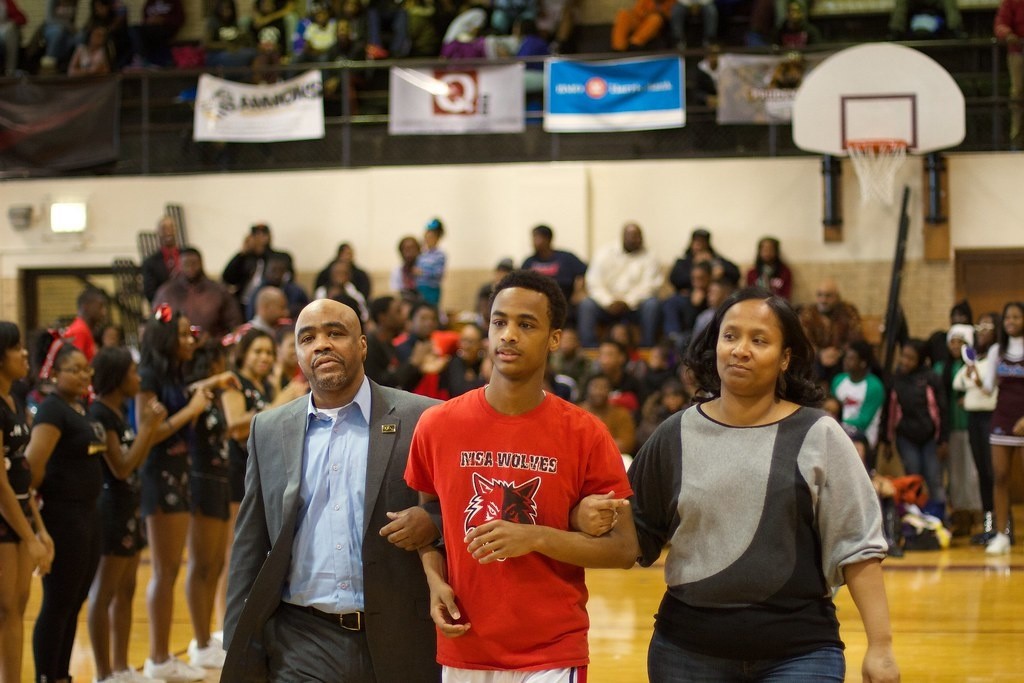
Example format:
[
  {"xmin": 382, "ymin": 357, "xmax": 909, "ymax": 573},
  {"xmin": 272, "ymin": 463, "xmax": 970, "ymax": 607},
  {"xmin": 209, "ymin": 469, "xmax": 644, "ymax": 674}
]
[{"xmin": 166, "ymin": 420, "xmax": 176, "ymax": 432}]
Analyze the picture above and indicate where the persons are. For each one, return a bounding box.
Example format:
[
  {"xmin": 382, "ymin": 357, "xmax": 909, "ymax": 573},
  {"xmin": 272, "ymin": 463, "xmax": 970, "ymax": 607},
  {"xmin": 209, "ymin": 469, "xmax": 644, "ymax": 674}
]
[
  {"xmin": 521, "ymin": 224, "xmax": 588, "ymax": 307},
  {"xmin": 86, "ymin": 345, "xmax": 166, "ymax": 683},
  {"xmin": 0, "ymin": 0, "xmax": 1024, "ymax": 150},
  {"xmin": 217, "ymin": 297, "xmax": 447, "ymax": 683},
  {"xmin": 742, "ymin": 236, "xmax": 793, "ymax": 302},
  {"xmin": 574, "ymin": 221, "xmax": 665, "ymax": 348},
  {"xmin": 568, "ymin": 284, "xmax": 902, "ymax": 683},
  {"xmin": 830, "ymin": 337, "xmax": 884, "ymax": 477},
  {"xmin": 150, "ymin": 247, "xmax": 242, "ymax": 336},
  {"xmin": 932, "ymin": 319, "xmax": 982, "ymax": 538},
  {"xmin": 885, "ymin": 338, "xmax": 949, "ymax": 507},
  {"xmin": 474, "ymin": 257, "xmax": 515, "ymax": 314},
  {"xmin": 0, "ymin": 217, "xmax": 1024, "ymax": 683},
  {"xmin": 312, "ymin": 242, "xmax": 369, "ymax": 300},
  {"xmin": 220, "ymin": 284, "xmax": 287, "ymax": 371},
  {"xmin": 403, "ymin": 269, "xmax": 638, "ymax": 683},
  {"xmin": 221, "ymin": 222, "xmax": 295, "ymax": 297}
]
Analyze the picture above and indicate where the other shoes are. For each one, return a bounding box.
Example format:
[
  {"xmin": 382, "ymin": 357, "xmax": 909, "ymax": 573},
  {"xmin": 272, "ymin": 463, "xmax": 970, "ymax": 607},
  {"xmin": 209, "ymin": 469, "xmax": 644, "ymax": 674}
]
[
  {"xmin": 970, "ymin": 524, "xmax": 996, "ymax": 545},
  {"xmin": 986, "ymin": 533, "xmax": 1012, "ymax": 556}
]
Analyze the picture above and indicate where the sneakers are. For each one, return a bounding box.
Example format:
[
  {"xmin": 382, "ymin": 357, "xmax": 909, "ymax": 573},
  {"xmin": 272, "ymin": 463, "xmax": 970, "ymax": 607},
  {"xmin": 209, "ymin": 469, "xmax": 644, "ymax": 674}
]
[
  {"xmin": 192, "ymin": 646, "xmax": 228, "ymax": 668},
  {"xmin": 98, "ymin": 666, "xmax": 167, "ymax": 683},
  {"xmin": 188, "ymin": 634, "xmax": 223, "ymax": 656},
  {"xmin": 143, "ymin": 654, "xmax": 206, "ymax": 682}
]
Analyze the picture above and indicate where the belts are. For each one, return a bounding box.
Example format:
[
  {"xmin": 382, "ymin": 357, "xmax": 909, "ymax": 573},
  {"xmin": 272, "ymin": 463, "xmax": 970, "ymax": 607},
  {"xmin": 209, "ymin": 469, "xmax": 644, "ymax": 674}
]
[{"xmin": 282, "ymin": 599, "xmax": 365, "ymax": 633}]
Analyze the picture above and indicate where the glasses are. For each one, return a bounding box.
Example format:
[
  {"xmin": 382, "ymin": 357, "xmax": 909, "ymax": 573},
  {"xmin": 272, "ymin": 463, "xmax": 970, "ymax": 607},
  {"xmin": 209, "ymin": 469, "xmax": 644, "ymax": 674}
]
[{"xmin": 51, "ymin": 364, "xmax": 94, "ymax": 378}]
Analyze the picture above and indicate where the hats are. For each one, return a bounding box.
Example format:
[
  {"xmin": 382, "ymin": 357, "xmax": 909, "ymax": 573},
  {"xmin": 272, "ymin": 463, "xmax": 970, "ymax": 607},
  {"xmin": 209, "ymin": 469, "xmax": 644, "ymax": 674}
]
[{"xmin": 946, "ymin": 323, "xmax": 975, "ymax": 349}]
